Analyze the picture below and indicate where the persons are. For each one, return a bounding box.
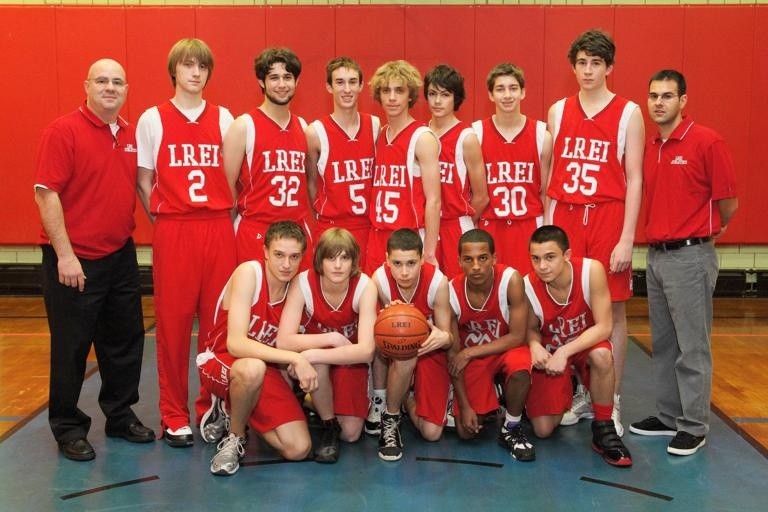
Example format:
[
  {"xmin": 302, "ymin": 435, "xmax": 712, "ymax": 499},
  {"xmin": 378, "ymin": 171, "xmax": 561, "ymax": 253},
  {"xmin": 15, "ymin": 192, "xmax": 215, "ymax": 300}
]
[
  {"xmin": 364, "ymin": 60, "xmax": 441, "ymax": 294},
  {"xmin": 441, "ymin": 230, "xmax": 537, "ymax": 461},
  {"xmin": 370, "ymin": 230, "xmax": 450, "ymax": 461},
  {"xmin": 630, "ymin": 69, "xmax": 736, "ymax": 458},
  {"xmin": 198, "ymin": 220, "xmax": 312, "ymax": 476},
  {"xmin": 304, "ymin": 53, "xmax": 381, "ymax": 272},
  {"xmin": 33, "ymin": 57, "xmax": 154, "ymax": 461},
  {"xmin": 473, "ymin": 64, "xmax": 553, "ymax": 276},
  {"xmin": 424, "ymin": 66, "xmax": 488, "ymax": 280},
  {"xmin": 222, "ymin": 46, "xmax": 312, "ymax": 266},
  {"xmin": 276, "ymin": 226, "xmax": 377, "ymax": 463},
  {"xmin": 524, "ymin": 225, "xmax": 632, "ymax": 468},
  {"xmin": 548, "ymin": 32, "xmax": 646, "ymax": 441},
  {"xmin": 135, "ymin": 38, "xmax": 237, "ymax": 448}
]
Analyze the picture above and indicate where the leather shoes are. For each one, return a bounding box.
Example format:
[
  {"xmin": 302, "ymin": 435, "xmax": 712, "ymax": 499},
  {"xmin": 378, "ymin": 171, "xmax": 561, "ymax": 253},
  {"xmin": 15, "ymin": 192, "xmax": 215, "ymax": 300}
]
[
  {"xmin": 58, "ymin": 437, "xmax": 95, "ymax": 461},
  {"xmin": 105, "ymin": 422, "xmax": 154, "ymax": 443}
]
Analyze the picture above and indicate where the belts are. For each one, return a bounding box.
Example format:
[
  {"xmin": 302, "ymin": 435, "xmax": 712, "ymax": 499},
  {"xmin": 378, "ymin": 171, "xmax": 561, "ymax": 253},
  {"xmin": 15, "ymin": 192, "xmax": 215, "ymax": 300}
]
[{"xmin": 649, "ymin": 236, "xmax": 712, "ymax": 250}]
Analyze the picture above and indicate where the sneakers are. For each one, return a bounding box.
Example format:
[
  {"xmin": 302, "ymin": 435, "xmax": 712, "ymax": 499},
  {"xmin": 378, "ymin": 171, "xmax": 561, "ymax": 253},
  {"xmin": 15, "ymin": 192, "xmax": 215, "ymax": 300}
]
[
  {"xmin": 364, "ymin": 395, "xmax": 386, "ymax": 436},
  {"xmin": 497, "ymin": 422, "xmax": 535, "ymax": 462},
  {"xmin": 666, "ymin": 431, "xmax": 705, "ymax": 456},
  {"xmin": 210, "ymin": 432, "xmax": 246, "ymax": 476},
  {"xmin": 199, "ymin": 392, "xmax": 230, "ymax": 444},
  {"xmin": 629, "ymin": 416, "xmax": 676, "ymax": 436},
  {"xmin": 314, "ymin": 424, "xmax": 340, "ymax": 463},
  {"xmin": 376, "ymin": 409, "xmax": 403, "ymax": 462},
  {"xmin": 558, "ymin": 384, "xmax": 632, "ymax": 466},
  {"xmin": 165, "ymin": 426, "xmax": 194, "ymax": 447}
]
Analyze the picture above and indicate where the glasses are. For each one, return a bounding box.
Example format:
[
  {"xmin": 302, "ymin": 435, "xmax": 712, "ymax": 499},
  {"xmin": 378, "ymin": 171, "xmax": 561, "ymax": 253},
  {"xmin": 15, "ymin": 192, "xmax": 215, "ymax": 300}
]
[{"xmin": 647, "ymin": 92, "xmax": 678, "ymax": 100}]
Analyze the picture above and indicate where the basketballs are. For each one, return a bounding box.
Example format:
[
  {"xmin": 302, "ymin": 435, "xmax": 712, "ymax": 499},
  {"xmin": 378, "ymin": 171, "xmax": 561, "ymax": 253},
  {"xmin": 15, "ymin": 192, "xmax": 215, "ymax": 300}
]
[{"xmin": 374, "ymin": 305, "xmax": 427, "ymax": 359}]
